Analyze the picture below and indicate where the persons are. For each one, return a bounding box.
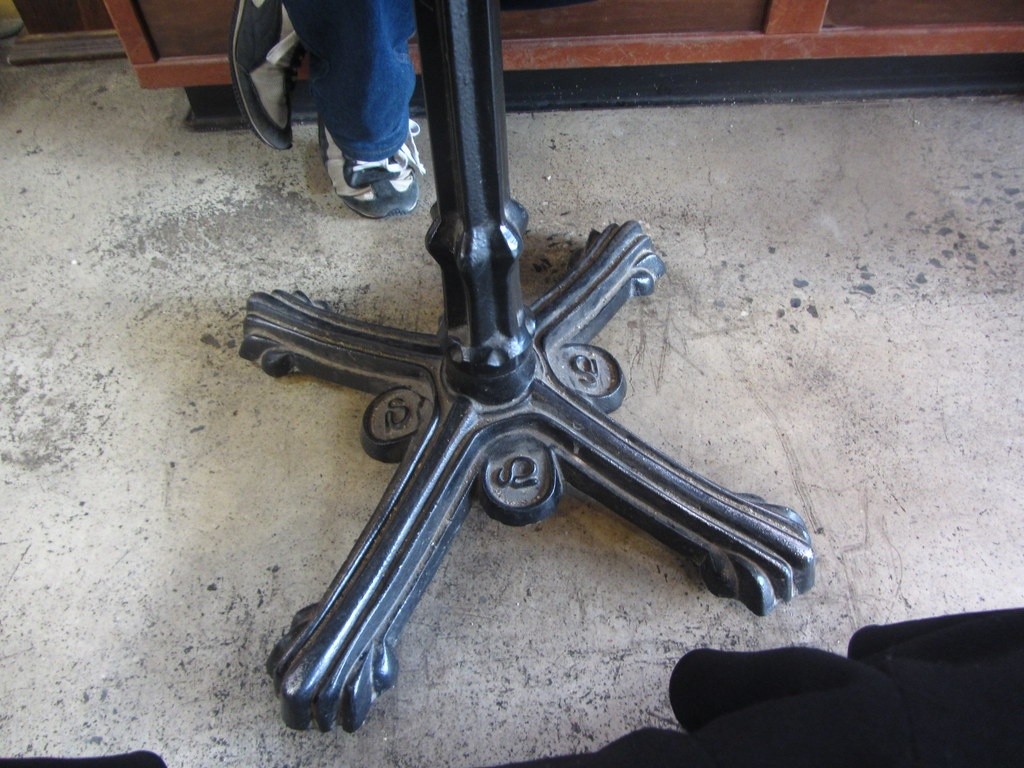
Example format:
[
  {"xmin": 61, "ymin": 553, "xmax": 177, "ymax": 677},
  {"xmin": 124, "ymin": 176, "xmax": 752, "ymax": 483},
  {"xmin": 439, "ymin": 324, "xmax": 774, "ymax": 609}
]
[{"xmin": 227, "ymin": 0, "xmax": 426, "ymax": 219}]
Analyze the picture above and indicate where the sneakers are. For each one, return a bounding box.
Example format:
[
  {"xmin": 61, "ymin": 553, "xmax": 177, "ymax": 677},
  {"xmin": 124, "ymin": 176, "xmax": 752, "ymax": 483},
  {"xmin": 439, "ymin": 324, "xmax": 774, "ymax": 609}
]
[
  {"xmin": 228, "ymin": 0, "xmax": 308, "ymax": 150},
  {"xmin": 316, "ymin": 95, "xmax": 426, "ymax": 219}
]
